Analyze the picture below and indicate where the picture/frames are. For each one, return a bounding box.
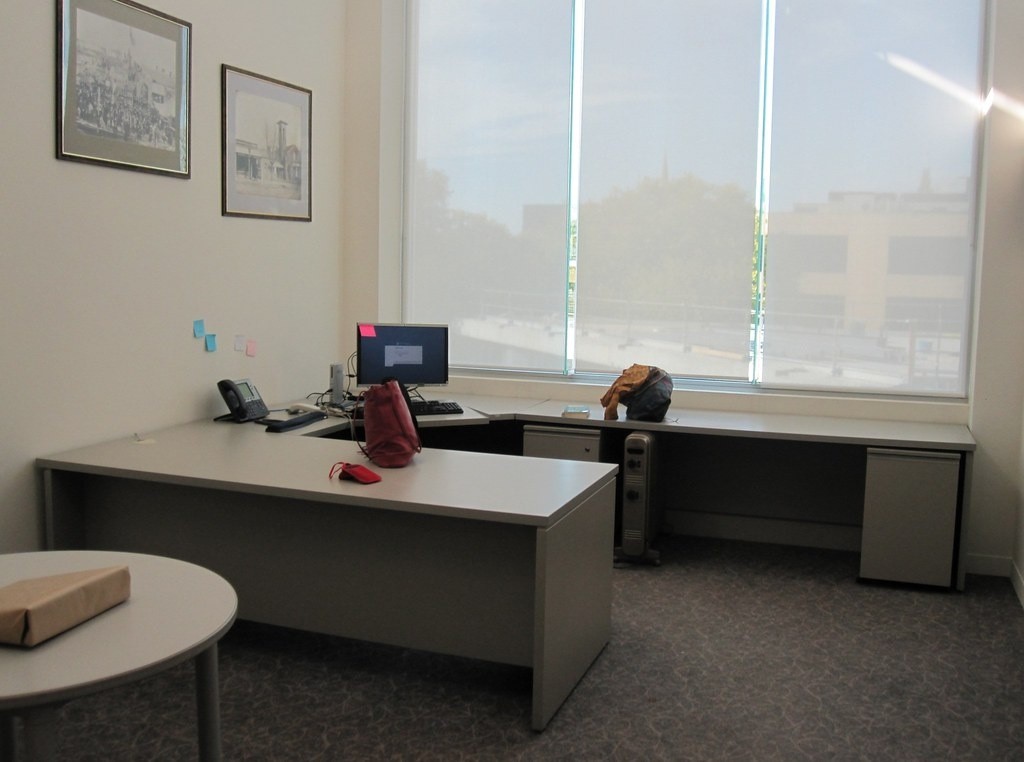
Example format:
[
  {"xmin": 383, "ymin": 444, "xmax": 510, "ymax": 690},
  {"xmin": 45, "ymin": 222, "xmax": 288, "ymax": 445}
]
[
  {"xmin": 219, "ymin": 61, "xmax": 313, "ymax": 223},
  {"xmin": 53, "ymin": 0, "xmax": 193, "ymax": 179}
]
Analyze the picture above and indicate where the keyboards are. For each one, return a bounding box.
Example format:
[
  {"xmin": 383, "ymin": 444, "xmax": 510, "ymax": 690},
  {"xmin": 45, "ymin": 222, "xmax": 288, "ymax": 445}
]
[{"xmin": 411, "ymin": 400, "xmax": 463, "ymax": 416}]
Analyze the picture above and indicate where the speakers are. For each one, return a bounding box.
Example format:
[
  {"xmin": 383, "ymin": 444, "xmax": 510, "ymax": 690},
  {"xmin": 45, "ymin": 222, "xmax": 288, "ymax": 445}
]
[{"xmin": 329, "ymin": 361, "xmax": 344, "ymax": 405}]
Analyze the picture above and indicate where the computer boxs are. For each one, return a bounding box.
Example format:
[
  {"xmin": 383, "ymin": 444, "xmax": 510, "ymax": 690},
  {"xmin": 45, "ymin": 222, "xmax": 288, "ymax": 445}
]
[{"xmin": 620, "ymin": 431, "xmax": 656, "ymax": 559}]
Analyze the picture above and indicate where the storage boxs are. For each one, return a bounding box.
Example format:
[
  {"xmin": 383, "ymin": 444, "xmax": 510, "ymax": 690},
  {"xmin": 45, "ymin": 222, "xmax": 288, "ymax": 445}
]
[{"xmin": 0, "ymin": 564, "xmax": 131, "ymax": 647}]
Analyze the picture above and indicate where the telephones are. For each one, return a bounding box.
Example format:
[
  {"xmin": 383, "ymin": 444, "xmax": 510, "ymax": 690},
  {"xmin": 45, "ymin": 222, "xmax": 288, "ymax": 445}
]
[{"xmin": 218, "ymin": 378, "xmax": 270, "ymax": 421}]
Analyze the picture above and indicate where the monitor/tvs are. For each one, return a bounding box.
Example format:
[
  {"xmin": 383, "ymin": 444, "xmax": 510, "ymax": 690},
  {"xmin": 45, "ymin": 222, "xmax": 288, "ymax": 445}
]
[{"xmin": 356, "ymin": 322, "xmax": 449, "ymax": 387}]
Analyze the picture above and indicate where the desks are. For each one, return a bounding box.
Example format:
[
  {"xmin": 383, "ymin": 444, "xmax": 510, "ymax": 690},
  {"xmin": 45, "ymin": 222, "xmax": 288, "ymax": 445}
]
[
  {"xmin": 0, "ymin": 550, "xmax": 238, "ymax": 762},
  {"xmin": 35, "ymin": 392, "xmax": 977, "ymax": 732}
]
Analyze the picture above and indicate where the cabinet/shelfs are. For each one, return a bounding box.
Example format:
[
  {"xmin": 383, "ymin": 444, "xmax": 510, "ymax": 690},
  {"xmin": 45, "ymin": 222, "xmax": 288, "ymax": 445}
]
[
  {"xmin": 522, "ymin": 423, "xmax": 602, "ymax": 464},
  {"xmin": 855, "ymin": 447, "xmax": 962, "ymax": 589}
]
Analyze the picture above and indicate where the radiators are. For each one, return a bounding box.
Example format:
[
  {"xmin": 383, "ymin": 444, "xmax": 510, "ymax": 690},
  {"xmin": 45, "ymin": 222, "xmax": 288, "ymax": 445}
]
[{"xmin": 615, "ymin": 431, "xmax": 674, "ymax": 567}]
